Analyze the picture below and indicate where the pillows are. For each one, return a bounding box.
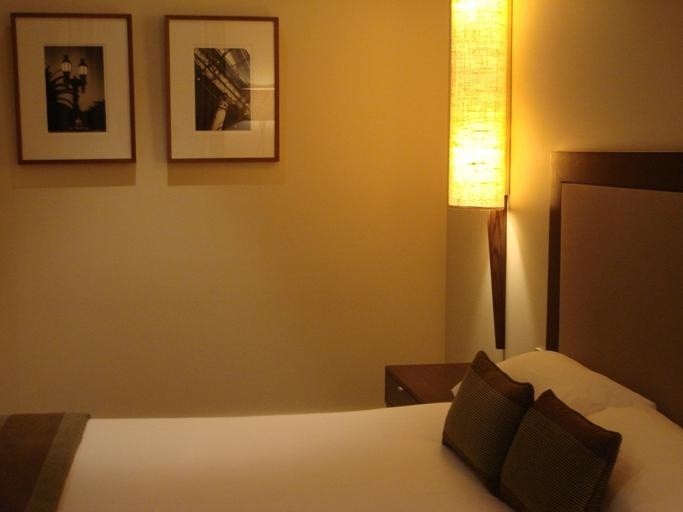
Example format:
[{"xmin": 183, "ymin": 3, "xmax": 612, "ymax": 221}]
[
  {"xmin": 583, "ymin": 405, "xmax": 682, "ymax": 510},
  {"xmin": 500, "ymin": 389, "xmax": 622, "ymax": 510},
  {"xmin": 452, "ymin": 347, "xmax": 656, "ymax": 416},
  {"xmin": 441, "ymin": 351, "xmax": 535, "ymax": 496}
]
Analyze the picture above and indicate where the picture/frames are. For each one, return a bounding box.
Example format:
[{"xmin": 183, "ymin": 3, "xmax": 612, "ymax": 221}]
[
  {"xmin": 166, "ymin": 16, "xmax": 280, "ymax": 162},
  {"xmin": 11, "ymin": 12, "xmax": 137, "ymax": 165}
]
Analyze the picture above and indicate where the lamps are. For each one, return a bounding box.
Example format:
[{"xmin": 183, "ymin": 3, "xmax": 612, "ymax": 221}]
[{"xmin": 444, "ymin": 2, "xmax": 509, "ymax": 349}]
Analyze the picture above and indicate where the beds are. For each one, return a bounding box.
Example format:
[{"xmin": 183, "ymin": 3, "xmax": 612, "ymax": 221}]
[{"xmin": 5, "ymin": 145, "xmax": 683, "ymax": 512}]
